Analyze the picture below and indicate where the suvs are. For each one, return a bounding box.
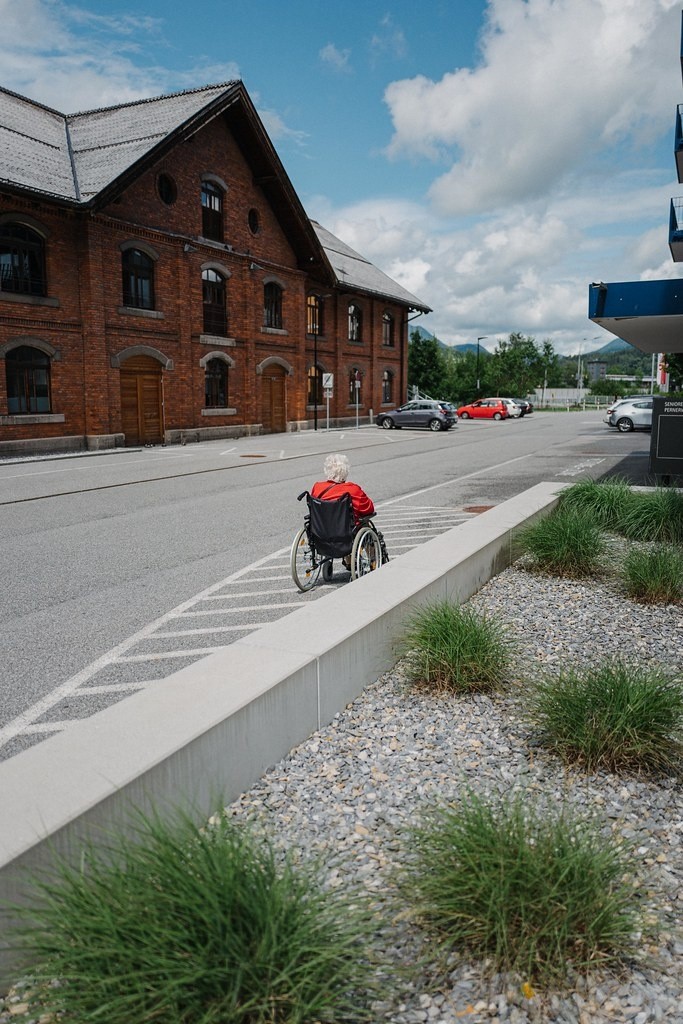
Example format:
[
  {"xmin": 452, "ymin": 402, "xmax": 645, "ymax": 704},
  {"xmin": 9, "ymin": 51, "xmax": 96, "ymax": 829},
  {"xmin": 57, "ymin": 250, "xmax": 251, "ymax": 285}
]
[{"xmin": 375, "ymin": 399, "xmax": 459, "ymax": 432}]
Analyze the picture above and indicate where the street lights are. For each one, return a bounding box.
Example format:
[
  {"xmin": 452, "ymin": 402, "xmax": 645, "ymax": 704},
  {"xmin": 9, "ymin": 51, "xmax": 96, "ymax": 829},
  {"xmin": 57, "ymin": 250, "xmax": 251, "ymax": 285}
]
[{"xmin": 476, "ymin": 336, "xmax": 488, "ymax": 401}]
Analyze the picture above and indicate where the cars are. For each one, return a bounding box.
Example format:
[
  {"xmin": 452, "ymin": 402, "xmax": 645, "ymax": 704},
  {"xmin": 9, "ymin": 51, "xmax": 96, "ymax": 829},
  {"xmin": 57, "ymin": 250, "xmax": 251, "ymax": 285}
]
[
  {"xmin": 603, "ymin": 394, "xmax": 664, "ymax": 427},
  {"xmin": 514, "ymin": 398, "xmax": 534, "ymax": 417},
  {"xmin": 499, "ymin": 398, "xmax": 522, "ymax": 417},
  {"xmin": 456, "ymin": 398, "xmax": 509, "ymax": 420},
  {"xmin": 610, "ymin": 400, "xmax": 653, "ymax": 432}
]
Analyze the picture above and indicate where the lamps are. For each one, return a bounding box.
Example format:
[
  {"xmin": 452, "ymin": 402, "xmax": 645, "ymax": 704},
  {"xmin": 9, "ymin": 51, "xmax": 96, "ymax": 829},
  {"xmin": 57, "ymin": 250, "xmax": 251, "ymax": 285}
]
[
  {"xmin": 184, "ymin": 243, "xmax": 199, "ymax": 254},
  {"xmin": 249, "ymin": 261, "xmax": 264, "ymax": 270}
]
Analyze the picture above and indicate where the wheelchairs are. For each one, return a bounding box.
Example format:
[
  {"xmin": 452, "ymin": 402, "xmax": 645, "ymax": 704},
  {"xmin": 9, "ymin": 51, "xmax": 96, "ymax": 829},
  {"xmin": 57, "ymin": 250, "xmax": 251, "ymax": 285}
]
[{"xmin": 290, "ymin": 491, "xmax": 388, "ymax": 594}]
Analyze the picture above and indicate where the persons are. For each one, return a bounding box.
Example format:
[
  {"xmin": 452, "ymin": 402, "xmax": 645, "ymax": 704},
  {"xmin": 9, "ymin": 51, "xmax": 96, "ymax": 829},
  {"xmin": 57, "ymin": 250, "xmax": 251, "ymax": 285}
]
[{"xmin": 308, "ymin": 455, "xmax": 374, "ymax": 566}]
